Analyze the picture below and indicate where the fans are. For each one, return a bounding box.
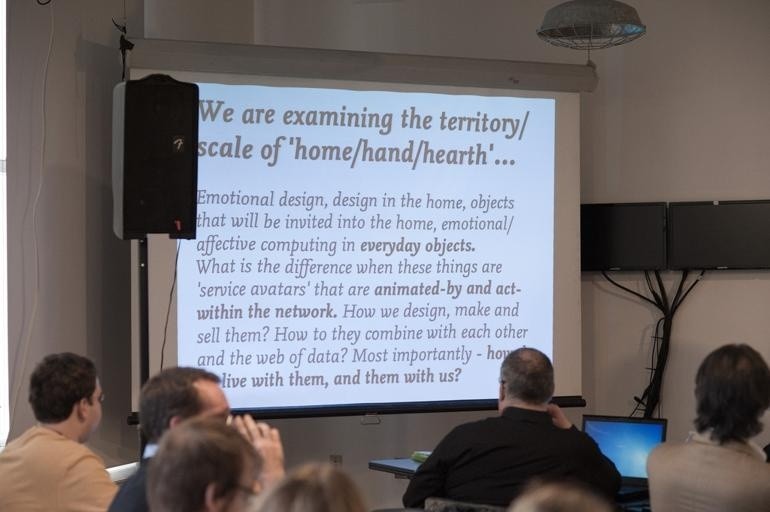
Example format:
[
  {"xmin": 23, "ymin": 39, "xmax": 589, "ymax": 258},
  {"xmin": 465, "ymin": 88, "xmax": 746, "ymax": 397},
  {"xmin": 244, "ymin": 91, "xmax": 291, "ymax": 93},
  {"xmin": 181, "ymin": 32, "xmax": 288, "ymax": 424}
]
[{"xmin": 535, "ymin": 0, "xmax": 645, "ymax": 52}]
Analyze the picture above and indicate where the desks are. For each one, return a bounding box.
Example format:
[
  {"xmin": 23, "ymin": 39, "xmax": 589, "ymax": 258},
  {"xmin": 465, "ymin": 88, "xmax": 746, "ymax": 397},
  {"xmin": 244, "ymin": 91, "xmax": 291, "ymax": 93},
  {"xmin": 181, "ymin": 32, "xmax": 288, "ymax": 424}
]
[{"xmin": 368, "ymin": 452, "xmax": 650, "ymax": 510}]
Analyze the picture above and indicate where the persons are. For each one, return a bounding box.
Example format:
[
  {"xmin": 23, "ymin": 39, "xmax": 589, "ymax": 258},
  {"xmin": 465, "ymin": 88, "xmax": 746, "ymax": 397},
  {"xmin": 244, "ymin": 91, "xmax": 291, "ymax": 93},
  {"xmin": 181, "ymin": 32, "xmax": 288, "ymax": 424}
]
[
  {"xmin": 108, "ymin": 367, "xmax": 365, "ymax": 511},
  {"xmin": 1, "ymin": 352, "xmax": 120, "ymax": 511},
  {"xmin": 646, "ymin": 344, "xmax": 770, "ymax": 511},
  {"xmin": 403, "ymin": 348, "xmax": 625, "ymax": 511}
]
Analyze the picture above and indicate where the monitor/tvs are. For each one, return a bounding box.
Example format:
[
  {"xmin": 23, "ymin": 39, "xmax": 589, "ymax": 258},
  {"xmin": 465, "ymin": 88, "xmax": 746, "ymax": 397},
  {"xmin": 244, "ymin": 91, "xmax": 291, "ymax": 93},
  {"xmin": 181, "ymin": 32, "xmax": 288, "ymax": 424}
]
[
  {"xmin": 668, "ymin": 199, "xmax": 770, "ymax": 270},
  {"xmin": 580, "ymin": 202, "xmax": 667, "ymax": 271}
]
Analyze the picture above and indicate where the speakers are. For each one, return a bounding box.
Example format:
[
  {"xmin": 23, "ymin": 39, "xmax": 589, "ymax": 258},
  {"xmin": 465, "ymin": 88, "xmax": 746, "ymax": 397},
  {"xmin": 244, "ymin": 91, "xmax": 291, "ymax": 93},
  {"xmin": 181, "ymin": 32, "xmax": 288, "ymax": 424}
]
[{"xmin": 111, "ymin": 74, "xmax": 199, "ymax": 242}]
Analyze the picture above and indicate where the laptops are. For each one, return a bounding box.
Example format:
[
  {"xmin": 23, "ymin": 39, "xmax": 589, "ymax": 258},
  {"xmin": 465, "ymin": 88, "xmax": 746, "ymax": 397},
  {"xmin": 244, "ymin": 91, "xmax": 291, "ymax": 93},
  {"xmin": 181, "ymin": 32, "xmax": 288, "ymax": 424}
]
[{"xmin": 581, "ymin": 414, "xmax": 668, "ymax": 504}]
